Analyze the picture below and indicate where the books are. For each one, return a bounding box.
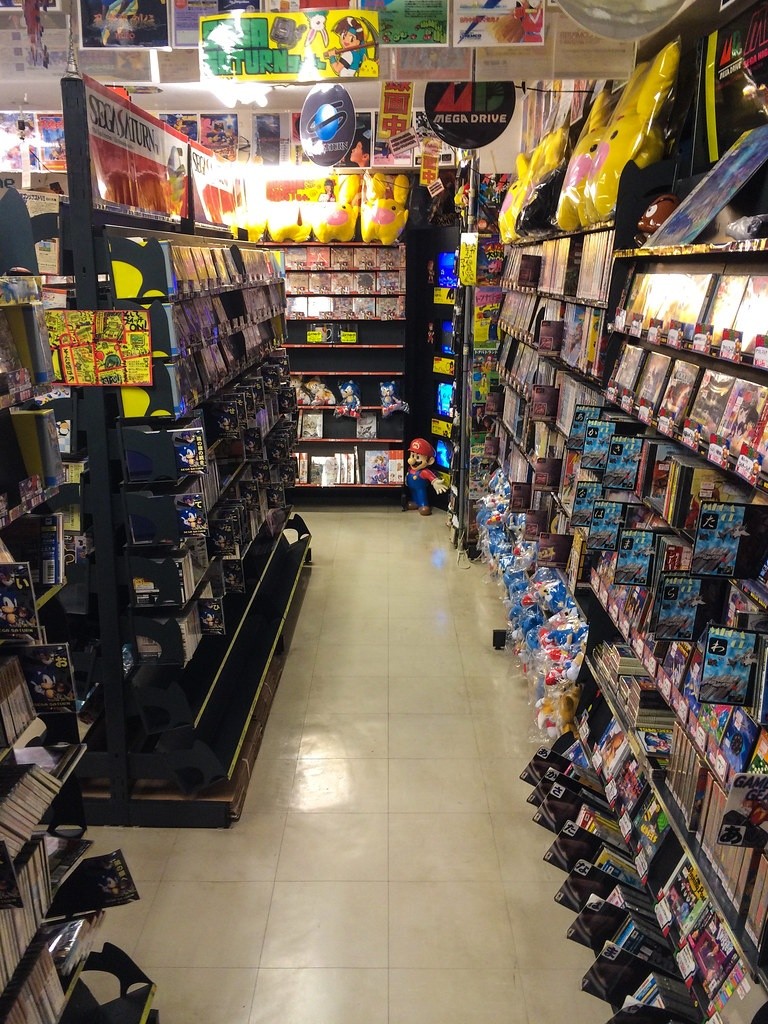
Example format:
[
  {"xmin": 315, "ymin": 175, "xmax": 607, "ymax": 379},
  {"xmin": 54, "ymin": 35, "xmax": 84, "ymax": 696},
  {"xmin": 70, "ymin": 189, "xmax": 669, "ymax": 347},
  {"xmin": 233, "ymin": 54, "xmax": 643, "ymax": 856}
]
[
  {"xmin": 356, "ymin": 411, "xmax": 376, "ymax": 439},
  {"xmin": 297, "ymin": 409, "xmax": 323, "ymax": 438},
  {"xmin": 496, "ymin": 230, "xmax": 768, "ymax": 1024},
  {"xmin": 291, "ymin": 445, "xmax": 404, "ymax": 485},
  {"xmin": 127, "ymin": 245, "xmax": 299, "ymax": 669},
  {"xmin": 0, "ymin": 310, "xmax": 63, "ymax": 1024}
]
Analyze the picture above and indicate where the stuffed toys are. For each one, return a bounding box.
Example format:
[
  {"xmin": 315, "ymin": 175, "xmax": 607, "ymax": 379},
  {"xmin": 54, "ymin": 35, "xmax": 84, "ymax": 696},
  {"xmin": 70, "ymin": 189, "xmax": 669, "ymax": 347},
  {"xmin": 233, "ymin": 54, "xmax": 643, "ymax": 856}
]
[
  {"xmin": 497, "ymin": 43, "xmax": 680, "ymax": 245},
  {"xmin": 339, "ymin": 380, "xmax": 363, "ymax": 419},
  {"xmin": 475, "ymin": 468, "xmax": 587, "ymax": 738},
  {"xmin": 289, "ymin": 376, "xmax": 336, "ymax": 406},
  {"xmin": 231, "ymin": 172, "xmax": 359, "ymax": 243},
  {"xmin": 379, "ymin": 381, "xmax": 410, "ymax": 417},
  {"xmin": 360, "ymin": 172, "xmax": 409, "ymax": 244}
]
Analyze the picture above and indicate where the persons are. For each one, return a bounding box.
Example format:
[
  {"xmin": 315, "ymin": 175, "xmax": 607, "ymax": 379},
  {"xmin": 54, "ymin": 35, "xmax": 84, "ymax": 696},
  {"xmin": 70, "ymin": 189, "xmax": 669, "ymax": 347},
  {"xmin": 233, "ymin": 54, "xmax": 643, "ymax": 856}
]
[
  {"xmin": 406, "ymin": 438, "xmax": 448, "ymax": 515},
  {"xmin": 473, "ymin": 406, "xmax": 484, "ymax": 431},
  {"xmin": 350, "ymin": 140, "xmax": 370, "ymax": 167}
]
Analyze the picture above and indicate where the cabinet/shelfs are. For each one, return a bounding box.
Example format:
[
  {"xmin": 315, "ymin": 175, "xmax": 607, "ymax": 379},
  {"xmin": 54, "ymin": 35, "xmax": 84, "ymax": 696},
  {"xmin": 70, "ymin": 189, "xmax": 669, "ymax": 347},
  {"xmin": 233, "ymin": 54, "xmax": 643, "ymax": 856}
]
[{"xmin": 0, "ymin": 73, "xmax": 768, "ymax": 1024}]
